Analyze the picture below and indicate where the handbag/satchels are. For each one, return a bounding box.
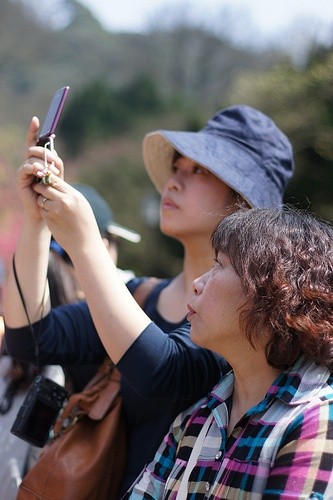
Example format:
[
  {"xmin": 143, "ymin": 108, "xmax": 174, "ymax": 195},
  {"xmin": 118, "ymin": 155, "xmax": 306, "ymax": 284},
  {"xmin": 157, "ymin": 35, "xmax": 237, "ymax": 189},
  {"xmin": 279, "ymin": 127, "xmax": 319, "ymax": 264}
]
[{"xmin": 14, "ymin": 278, "xmax": 162, "ymax": 500}]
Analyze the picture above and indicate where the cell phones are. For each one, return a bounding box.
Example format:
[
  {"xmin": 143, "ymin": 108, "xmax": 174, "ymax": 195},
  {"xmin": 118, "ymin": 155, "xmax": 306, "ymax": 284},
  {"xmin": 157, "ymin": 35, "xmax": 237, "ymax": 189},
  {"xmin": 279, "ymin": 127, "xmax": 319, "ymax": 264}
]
[{"xmin": 35, "ymin": 84, "xmax": 70, "ymax": 194}]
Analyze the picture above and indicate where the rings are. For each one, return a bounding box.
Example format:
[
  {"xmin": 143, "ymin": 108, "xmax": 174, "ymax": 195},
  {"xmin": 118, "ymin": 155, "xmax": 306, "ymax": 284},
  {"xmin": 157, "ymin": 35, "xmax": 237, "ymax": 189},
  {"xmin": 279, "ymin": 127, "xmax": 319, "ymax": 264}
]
[{"xmin": 41, "ymin": 198, "xmax": 49, "ymax": 208}]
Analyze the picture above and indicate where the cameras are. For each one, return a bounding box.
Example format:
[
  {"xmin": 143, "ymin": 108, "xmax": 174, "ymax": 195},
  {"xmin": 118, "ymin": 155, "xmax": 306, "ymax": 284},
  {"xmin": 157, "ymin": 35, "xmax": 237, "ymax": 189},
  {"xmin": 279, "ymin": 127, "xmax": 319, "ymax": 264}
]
[{"xmin": 9, "ymin": 374, "xmax": 69, "ymax": 448}]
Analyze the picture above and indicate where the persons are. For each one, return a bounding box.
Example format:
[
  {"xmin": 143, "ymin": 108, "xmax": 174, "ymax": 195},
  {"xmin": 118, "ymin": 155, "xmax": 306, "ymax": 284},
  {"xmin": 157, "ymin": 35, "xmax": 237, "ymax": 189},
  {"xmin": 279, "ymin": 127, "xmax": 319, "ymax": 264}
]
[
  {"xmin": 3, "ymin": 105, "xmax": 295, "ymax": 500},
  {"xmin": 128, "ymin": 209, "xmax": 333, "ymax": 500},
  {"xmin": 0, "ymin": 183, "xmax": 141, "ymax": 500}
]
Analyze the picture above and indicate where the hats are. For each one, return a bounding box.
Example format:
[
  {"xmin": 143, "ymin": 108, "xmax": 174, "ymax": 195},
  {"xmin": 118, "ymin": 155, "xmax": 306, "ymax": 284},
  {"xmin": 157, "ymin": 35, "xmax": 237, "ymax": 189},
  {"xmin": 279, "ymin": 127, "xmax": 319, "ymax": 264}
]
[
  {"xmin": 64, "ymin": 180, "xmax": 142, "ymax": 243},
  {"xmin": 142, "ymin": 106, "xmax": 296, "ymax": 214}
]
